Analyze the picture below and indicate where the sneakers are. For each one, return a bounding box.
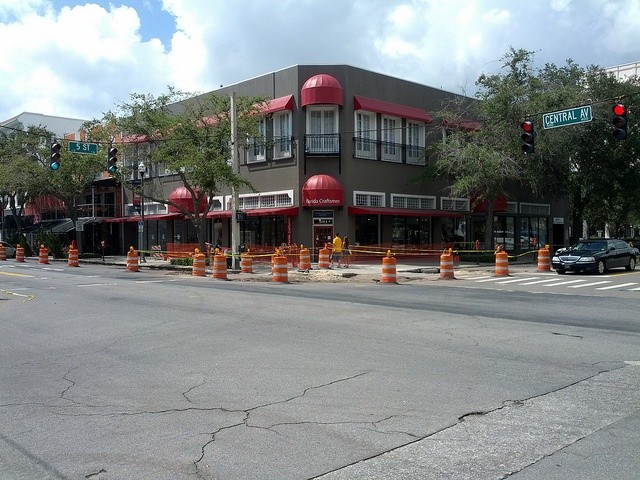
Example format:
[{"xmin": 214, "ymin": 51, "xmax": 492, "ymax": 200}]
[
  {"xmin": 328, "ymin": 264, "xmax": 334, "ymax": 268},
  {"xmin": 336, "ymin": 265, "xmax": 340, "ymax": 268}
]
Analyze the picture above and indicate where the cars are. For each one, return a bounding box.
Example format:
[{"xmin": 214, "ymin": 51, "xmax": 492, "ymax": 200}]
[
  {"xmin": 0, "ymin": 241, "xmax": 16, "ymax": 258},
  {"xmin": 551, "ymin": 238, "xmax": 639, "ymax": 274}
]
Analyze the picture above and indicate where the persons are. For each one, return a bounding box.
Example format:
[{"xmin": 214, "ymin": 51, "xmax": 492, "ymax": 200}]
[
  {"xmin": 343, "ymin": 236, "xmax": 350, "ymax": 268},
  {"xmin": 329, "ymin": 233, "xmax": 343, "ymax": 269}
]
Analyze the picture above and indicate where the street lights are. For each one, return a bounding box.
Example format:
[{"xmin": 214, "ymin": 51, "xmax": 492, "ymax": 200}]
[{"xmin": 138, "ymin": 161, "xmax": 147, "ymax": 262}]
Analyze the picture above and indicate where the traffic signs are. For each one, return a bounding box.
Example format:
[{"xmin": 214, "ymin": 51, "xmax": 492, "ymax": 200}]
[
  {"xmin": 69, "ymin": 142, "xmax": 98, "ymax": 155},
  {"xmin": 542, "ymin": 105, "xmax": 592, "ymax": 129}
]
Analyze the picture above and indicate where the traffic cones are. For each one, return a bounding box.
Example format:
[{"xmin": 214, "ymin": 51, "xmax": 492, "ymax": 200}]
[
  {"xmin": 239, "ymin": 255, "xmax": 254, "ymax": 275},
  {"xmin": 535, "ymin": 248, "xmax": 555, "ymax": 272},
  {"xmin": 126, "ymin": 250, "xmax": 140, "ymax": 272},
  {"xmin": 16, "ymin": 247, "xmax": 25, "ymax": 262},
  {"xmin": 269, "ymin": 253, "xmax": 285, "ymax": 275},
  {"xmin": 314, "ymin": 249, "xmax": 332, "ymax": 270},
  {"xmin": 491, "ymin": 251, "xmax": 514, "ymax": 277},
  {"xmin": 297, "ymin": 250, "xmax": 313, "ymax": 272},
  {"xmin": 208, "ymin": 255, "xmax": 232, "ymax": 281},
  {"xmin": 267, "ymin": 257, "xmax": 293, "ymax": 285},
  {"xmin": 376, "ymin": 257, "xmax": 399, "ymax": 285},
  {"xmin": 192, "ymin": 253, "xmax": 207, "ymax": 277},
  {"xmin": 38, "ymin": 247, "xmax": 50, "ymax": 264},
  {"xmin": 436, "ymin": 253, "xmax": 459, "ymax": 281},
  {"xmin": 67, "ymin": 249, "xmax": 80, "ymax": 267},
  {"xmin": 0, "ymin": 247, "xmax": 7, "ymax": 261}
]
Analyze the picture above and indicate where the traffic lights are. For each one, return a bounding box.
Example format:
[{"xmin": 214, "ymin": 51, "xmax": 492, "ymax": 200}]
[
  {"xmin": 521, "ymin": 121, "xmax": 535, "ymax": 154},
  {"xmin": 613, "ymin": 104, "xmax": 626, "ymax": 140},
  {"xmin": 108, "ymin": 147, "xmax": 118, "ymax": 173},
  {"xmin": 50, "ymin": 142, "xmax": 61, "ymax": 170}
]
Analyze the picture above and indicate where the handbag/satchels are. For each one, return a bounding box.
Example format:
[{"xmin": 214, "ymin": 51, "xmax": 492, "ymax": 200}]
[{"xmin": 349, "ymin": 251, "xmax": 352, "ymax": 255}]
